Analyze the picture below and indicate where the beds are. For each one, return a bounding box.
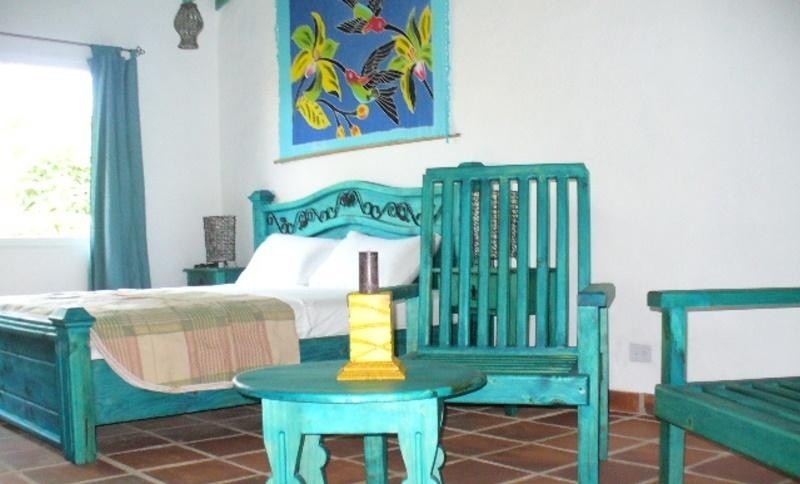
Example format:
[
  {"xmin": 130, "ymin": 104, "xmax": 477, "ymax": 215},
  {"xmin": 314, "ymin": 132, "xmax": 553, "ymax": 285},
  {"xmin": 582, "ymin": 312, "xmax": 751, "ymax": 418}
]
[{"xmin": 0, "ymin": 162, "xmax": 491, "ymax": 467}]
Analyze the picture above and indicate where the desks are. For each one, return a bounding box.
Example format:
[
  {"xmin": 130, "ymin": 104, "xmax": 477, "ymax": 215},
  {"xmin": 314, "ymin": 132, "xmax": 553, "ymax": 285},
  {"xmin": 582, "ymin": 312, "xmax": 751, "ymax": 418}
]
[{"xmin": 234, "ymin": 358, "xmax": 486, "ymax": 484}]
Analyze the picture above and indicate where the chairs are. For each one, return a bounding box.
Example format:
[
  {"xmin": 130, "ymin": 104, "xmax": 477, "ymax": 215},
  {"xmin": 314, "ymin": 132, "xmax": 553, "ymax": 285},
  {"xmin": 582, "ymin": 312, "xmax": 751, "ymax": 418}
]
[
  {"xmin": 648, "ymin": 291, "xmax": 798, "ymax": 482},
  {"xmin": 364, "ymin": 164, "xmax": 615, "ymax": 484}
]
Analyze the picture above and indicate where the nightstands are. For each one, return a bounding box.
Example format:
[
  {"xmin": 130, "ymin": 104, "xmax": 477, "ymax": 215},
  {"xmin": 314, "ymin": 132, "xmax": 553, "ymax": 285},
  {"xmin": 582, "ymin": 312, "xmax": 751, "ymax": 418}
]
[
  {"xmin": 431, "ymin": 265, "xmax": 566, "ymax": 418},
  {"xmin": 182, "ymin": 266, "xmax": 245, "ymax": 285}
]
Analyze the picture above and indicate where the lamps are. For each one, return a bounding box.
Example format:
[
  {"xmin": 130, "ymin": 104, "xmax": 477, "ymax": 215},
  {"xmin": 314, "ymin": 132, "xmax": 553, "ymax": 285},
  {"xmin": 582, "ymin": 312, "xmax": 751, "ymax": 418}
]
[{"xmin": 202, "ymin": 216, "xmax": 236, "ymax": 266}]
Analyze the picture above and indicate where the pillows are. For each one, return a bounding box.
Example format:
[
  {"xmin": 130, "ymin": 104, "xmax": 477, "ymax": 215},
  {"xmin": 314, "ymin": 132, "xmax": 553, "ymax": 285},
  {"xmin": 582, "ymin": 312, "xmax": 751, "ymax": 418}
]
[{"xmin": 235, "ymin": 229, "xmax": 441, "ymax": 297}]
